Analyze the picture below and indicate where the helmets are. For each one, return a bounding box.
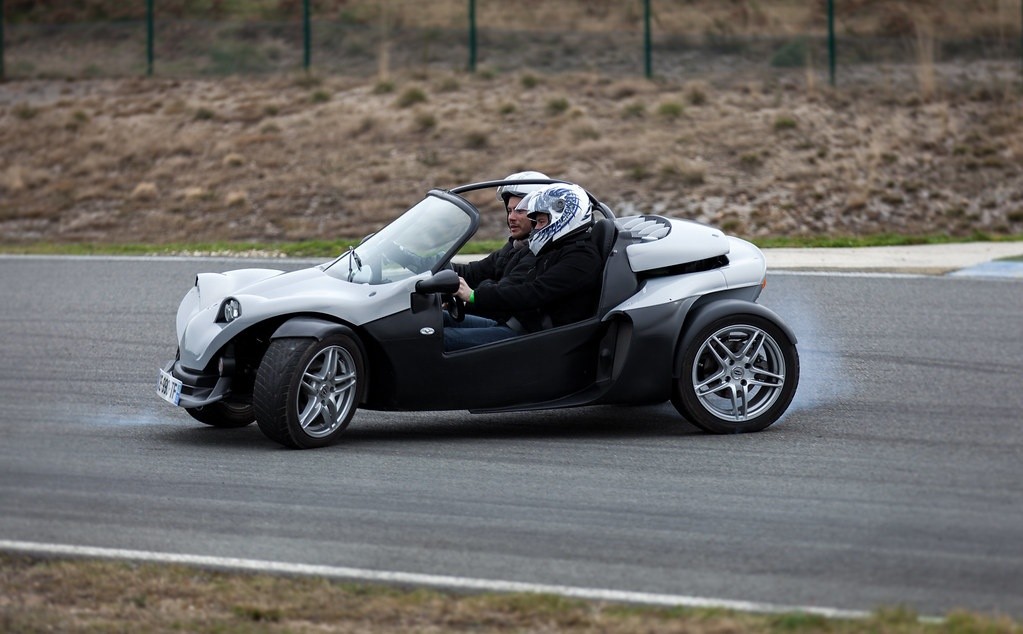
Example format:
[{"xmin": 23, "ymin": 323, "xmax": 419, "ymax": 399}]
[
  {"xmin": 502, "ymin": 172, "xmax": 551, "ymax": 229},
  {"xmin": 526, "ymin": 182, "xmax": 592, "ymax": 256}
]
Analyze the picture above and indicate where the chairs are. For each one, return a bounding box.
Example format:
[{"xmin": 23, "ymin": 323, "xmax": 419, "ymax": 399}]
[{"xmin": 591, "ymin": 218, "xmax": 616, "ymax": 280}]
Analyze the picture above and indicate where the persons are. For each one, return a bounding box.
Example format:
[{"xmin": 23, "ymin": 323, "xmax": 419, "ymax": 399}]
[{"xmin": 357, "ymin": 169, "xmax": 605, "ymax": 351}]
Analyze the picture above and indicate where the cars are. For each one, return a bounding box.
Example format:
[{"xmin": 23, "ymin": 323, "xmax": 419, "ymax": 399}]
[{"xmin": 156, "ymin": 179, "xmax": 800, "ymax": 449}]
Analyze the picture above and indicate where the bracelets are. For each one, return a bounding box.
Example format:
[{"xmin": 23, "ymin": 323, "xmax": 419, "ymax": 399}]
[{"xmin": 469, "ymin": 290, "xmax": 476, "ymax": 304}]
